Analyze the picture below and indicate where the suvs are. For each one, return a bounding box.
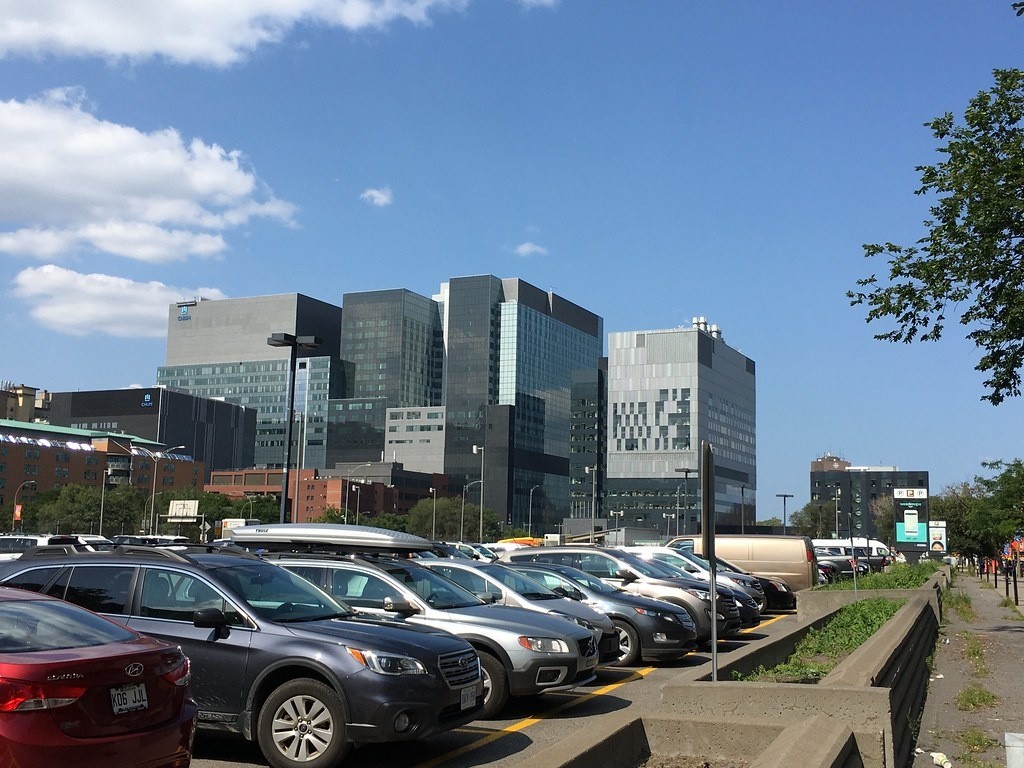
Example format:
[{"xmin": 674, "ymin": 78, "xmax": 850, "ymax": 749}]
[
  {"xmin": 0, "ymin": 532, "xmax": 95, "ymax": 562},
  {"xmin": 108, "ymin": 535, "xmax": 189, "ymax": 548},
  {"xmin": 500, "ymin": 545, "xmax": 711, "ymax": 643},
  {"xmin": 169, "ymin": 553, "xmax": 600, "ymax": 722},
  {"xmin": 72, "ymin": 535, "xmax": 115, "ymax": 554},
  {"xmin": 0, "ymin": 545, "xmax": 485, "ymax": 768}
]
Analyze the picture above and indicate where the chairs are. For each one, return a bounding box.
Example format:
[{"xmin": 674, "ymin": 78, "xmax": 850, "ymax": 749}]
[
  {"xmin": 188, "ymin": 579, "xmax": 218, "ymax": 606},
  {"xmin": 111, "ymin": 570, "xmax": 133, "ymax": 606},
  {"xmin": 334, "ymin": 574, "xmax": 348, "ymax": 596},
  {"xmin": 450, "ymin": 570, "xmax": 476, "ymax": 596},
  {"xmin": 142, "ymin": 577, "xmax": 169, "ymax": 607}
]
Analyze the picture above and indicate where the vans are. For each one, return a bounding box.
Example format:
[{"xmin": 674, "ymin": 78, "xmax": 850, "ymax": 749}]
[
  {"xmin": 811, "ymin": 539, "xmax": 893, "ymax": 573},
  {"xmin": 663, "ymin": 533, "xmax": 818, "ymax": 593}
]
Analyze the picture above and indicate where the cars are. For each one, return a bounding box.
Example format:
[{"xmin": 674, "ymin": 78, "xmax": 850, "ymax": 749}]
[
  {"xmin": 623, "ymin": 544, "xmax": 796, "ymax": 633},
  {"xmin": 813, "ymin": 548, "xmax": 868, "ymax": 585},
  {"xmin": 497, "ymin": 563, "xmax": 698, "ymax": 666},
  {"xmin": 0, "ymin": 587, "xmax": 198, "ymax": 768},
  {"xmin": 344, "ymin": 558, "xmax": 618, "ymax": 669}
]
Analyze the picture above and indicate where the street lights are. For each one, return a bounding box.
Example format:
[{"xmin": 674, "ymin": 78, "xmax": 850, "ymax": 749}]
[
  {"xmin": 12, "ymin": 480, "xmax": 36, "ymax": 532},
  {"xmin": 129, "ymin": 445, "xmax": 186, "ymax": 536},
  {"xmin": 240, "ymin": 494, "xmax": 257, "ymax": 520},
  {"xmin": 776, "ymin": 494, "xmax": 794, "ymax": 536},
  {"xmin": 143, "ymin": 492, "xmax": 163, "ymax": 535},
  {"xmin": 674, "ymin": 468, "xmax": 700, "ymax": 535},
  {"xmin": 345, "ymin": 463, "xmax": 371, "ymax": 524}
]
